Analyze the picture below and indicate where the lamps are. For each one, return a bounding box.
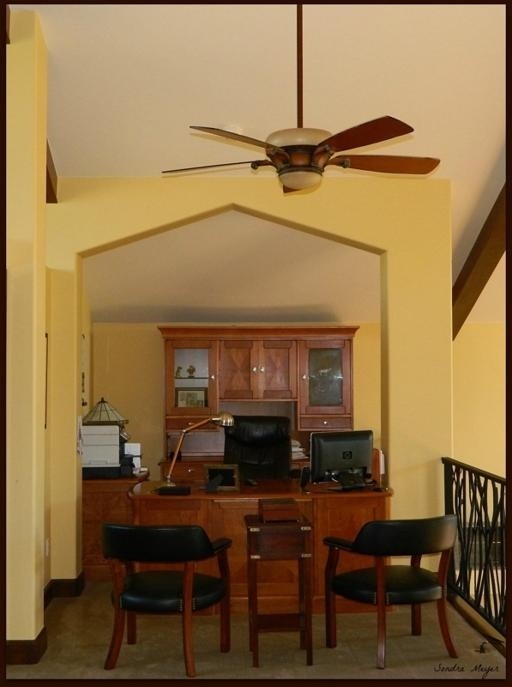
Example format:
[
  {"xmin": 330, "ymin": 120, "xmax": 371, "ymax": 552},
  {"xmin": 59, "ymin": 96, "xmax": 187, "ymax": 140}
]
[
  {"xmin": 83, "ymin": 397, "xmax": 129, "ymax": 427},
  {"xmin": 268, "ymin": 126, "xmax": 329, "ymax": 194},
  {"xmin": 157, "ymin": 412, "xmax": 235, "ymax": 487}
]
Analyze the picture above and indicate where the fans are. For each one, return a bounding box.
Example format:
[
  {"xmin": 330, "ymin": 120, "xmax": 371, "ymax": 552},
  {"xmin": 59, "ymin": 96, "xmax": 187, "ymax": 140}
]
[{"xmin": 158, "ymin": 4, "xmax": 449, "ymax": 176}]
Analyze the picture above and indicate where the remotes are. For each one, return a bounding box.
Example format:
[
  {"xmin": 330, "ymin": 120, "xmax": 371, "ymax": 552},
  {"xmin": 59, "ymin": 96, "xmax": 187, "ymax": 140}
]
[{"xmin": 245, "ymin": 478, "xmax": 258, "ymax": 486}]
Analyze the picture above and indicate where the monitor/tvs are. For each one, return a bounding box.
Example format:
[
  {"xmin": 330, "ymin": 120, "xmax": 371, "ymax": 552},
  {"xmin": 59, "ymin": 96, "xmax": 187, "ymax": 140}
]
[{"xmin": 309, "ymin": 429, "xmax": 374, "ymax": 492}]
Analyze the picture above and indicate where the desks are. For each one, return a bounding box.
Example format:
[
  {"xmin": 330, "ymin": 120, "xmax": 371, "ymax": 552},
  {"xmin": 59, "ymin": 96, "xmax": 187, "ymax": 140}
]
[
  {"xmin": 245, "ymin": 515, "xmax": 320, "ymax": 668},
  {"xmin": 129, "ymin": 477, "xmax": 394, "ymax": 616},
  {"xmin": 82, "ymin": 468, "xmax": 151, "ymax": 587}
]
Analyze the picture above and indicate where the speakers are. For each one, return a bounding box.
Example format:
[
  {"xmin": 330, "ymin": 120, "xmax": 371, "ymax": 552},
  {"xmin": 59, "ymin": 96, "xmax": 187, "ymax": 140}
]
[{"xmin": 300, "ymin": 467, "xmax": 310, "ymax": 494}]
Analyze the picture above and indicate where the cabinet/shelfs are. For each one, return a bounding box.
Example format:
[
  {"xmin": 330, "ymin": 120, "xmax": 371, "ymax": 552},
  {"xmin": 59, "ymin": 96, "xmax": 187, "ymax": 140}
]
[{"xmin": 156, "ymin": 322, "xmax": 361, "ymax": 480}]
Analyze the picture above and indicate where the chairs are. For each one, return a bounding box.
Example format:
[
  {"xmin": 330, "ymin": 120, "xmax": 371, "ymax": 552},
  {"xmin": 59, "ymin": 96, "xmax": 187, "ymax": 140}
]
[
  {"xmin": 99, "ymin": 517, "xmax": 234, "ymax": 677},
  {"xmin": 323, "ymin": 514, "xmax": 462, "ymax": 670}
]
